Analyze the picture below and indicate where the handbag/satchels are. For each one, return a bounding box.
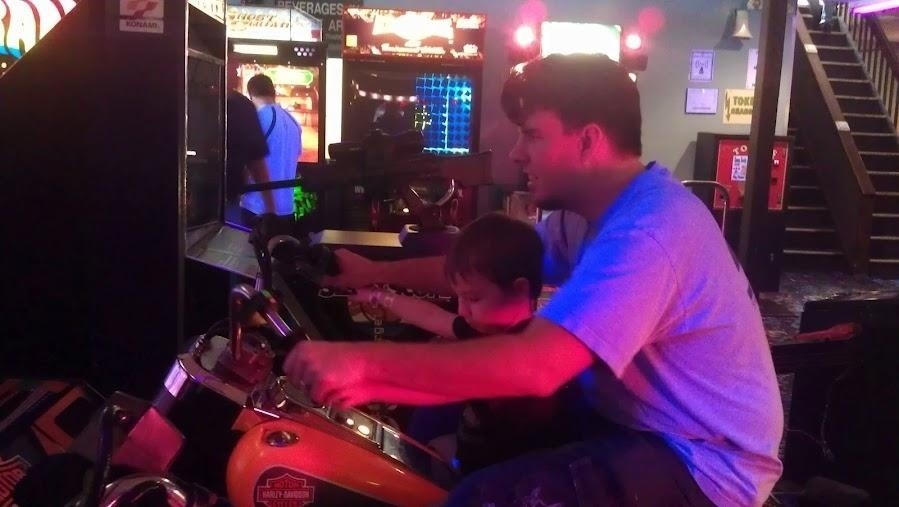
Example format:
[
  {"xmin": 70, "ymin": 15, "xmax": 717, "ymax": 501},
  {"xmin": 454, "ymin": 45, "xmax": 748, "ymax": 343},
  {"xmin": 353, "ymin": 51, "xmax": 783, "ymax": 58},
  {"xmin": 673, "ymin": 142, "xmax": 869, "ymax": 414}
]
[{"xmin": 369, "ymin": 286, "xmax": 395, "ymax": 312}]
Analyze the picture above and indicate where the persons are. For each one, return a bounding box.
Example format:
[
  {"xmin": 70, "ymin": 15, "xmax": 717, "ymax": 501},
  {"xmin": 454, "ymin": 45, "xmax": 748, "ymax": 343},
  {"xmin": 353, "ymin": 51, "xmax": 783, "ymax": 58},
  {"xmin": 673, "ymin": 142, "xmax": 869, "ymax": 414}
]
[
  {"xmin": 283, "ymin": 54, "xmax": 785, "ymax": 507},
  {"xmin": 343, "ymin": 213, "xmax": 576, "ymax": 478},
  {"xmin": 237, "ymin": 74, "xmax": 302, "ymax": 232},
  {"xmin": 228, "ymin": 54, "xmax": 276, "ymax": 215}
]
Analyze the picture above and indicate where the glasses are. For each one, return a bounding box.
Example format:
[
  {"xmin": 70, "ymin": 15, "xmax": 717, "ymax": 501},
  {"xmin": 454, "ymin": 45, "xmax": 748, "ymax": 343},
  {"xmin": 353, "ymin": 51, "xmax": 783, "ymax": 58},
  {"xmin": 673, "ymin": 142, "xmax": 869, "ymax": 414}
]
[{"xmin": 729, "ymin": 7, "xmax": 754, "ymax": 40}]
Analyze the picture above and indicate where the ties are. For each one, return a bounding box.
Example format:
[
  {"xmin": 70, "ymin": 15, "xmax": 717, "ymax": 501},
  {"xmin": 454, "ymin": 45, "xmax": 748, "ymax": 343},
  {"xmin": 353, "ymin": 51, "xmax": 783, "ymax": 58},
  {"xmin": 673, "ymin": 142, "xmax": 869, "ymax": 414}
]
[{"xmin": 187, "ymin": 48, "xmax": 224, "ymax": 230}]
